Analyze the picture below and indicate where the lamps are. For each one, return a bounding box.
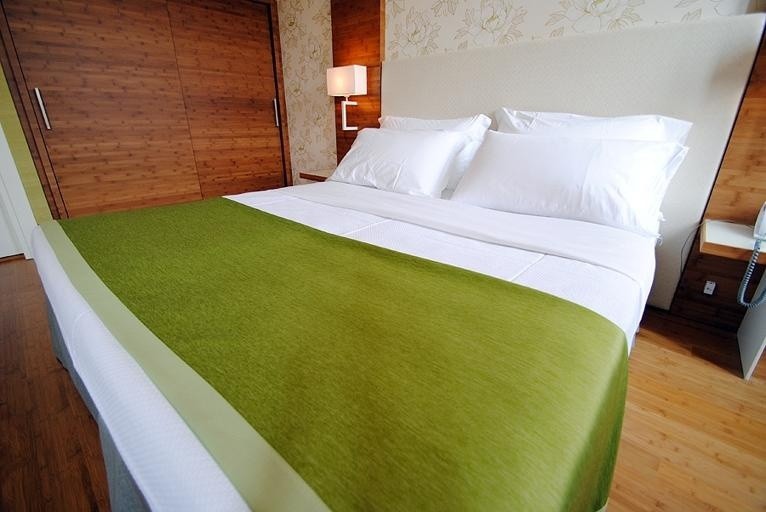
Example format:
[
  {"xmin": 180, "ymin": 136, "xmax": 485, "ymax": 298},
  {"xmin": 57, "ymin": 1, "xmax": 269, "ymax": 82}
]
[{"xmin": 326, "ymin": 65, "xmax": 367, "ymax": 131}]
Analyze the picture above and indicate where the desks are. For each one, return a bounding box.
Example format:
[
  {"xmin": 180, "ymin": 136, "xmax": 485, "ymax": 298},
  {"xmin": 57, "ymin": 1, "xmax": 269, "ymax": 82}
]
[
  {"xmin": 298, "ymin": 168, "xmax": 337, "ymax": 181},
  {"xmin": 698, "ymin": 217, "xmax": 766, "ymax": 265}
]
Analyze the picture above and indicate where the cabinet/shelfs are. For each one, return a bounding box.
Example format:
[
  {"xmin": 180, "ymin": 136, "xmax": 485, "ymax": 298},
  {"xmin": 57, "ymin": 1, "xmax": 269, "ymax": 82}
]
[{"xmin": 0, "ymin": 2, "xmax": 292, "ymax": 222}]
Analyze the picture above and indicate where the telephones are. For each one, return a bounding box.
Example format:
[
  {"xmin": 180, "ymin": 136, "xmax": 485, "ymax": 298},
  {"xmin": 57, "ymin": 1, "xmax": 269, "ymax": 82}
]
[{"xmin": 752, "ymin": 201, "xmax": 766, "ymax": 241}]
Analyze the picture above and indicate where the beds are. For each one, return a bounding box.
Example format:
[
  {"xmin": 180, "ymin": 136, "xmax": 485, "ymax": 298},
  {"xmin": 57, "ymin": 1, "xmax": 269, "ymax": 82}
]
[{"xmin": 30, "ymin": 179, "xmax": 662, "ymax": 508}]
